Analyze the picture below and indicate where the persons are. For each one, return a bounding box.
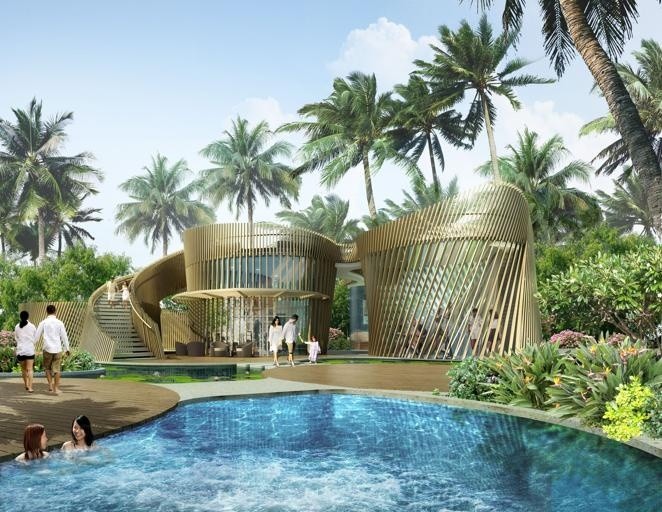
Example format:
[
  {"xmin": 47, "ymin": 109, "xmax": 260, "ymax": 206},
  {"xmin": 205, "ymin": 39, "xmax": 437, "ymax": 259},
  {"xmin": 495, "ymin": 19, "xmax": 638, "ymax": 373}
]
[
  {"xmin": 60, "ymin": 415, "xmax": 101, "ymax": 455},
  {"xmin": 13, "ymin": 422, "xmax": 49, "ymax": 463},
  {"xmin": 304, "ymin": 333, "xmax": 322, "ymax": 365},
  {"xmin": 281, "ymin": 314, "xmax": 304, "ymax": 366},
  {"xmin": 381, "ymin": 302, "xmax": 502, "ymax": 359},
  {"xmin": 13, "ymin": 310, "xmax": 37, "ymax": 392},
  {"xmin": 268, "ymin": 315, "xmax": 282, "ymax": 366},
  {"xmin": 106, "ymin": 277, "xmax": 118, "ymax": 309},
  {"xmin": 33, "ymin": 304, "xmax": 71, "ymax": 392},
  {"xmin": 119, "ymin": 280, "xmax": 131, "ymax": 309}
]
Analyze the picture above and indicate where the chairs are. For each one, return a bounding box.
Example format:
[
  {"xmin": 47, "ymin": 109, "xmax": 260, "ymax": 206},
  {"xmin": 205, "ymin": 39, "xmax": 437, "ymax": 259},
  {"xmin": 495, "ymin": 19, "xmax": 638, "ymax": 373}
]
[{"xmin": 174, "ymin": 340, "xmax": 255, "ymax": 357}]
